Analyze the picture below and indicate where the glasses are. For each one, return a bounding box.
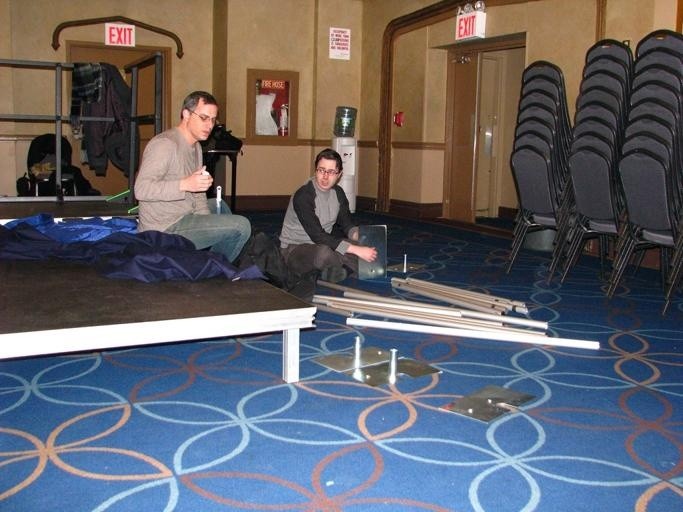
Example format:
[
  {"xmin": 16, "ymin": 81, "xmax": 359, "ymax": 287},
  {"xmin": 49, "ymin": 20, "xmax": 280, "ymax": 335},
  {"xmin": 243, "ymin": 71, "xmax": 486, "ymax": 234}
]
[
  {"xmin": 316, "ymin": 167, "xmax": 338, "ymax": 176},
  {"xmin": 189, "ymin": 109, "xmax": 217, "ymax": 123}
]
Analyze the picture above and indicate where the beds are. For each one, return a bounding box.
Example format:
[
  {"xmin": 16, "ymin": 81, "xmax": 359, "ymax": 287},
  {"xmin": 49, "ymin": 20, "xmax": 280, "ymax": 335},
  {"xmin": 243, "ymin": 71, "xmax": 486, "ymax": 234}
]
[{"xmin": 0, "ymin": 223, "xmax": 318, "ymax": 384}]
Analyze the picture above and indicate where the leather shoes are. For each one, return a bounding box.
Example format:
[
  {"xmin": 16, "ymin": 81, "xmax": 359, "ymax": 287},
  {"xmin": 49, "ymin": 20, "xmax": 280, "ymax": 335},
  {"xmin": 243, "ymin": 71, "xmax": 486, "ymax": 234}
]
[{"xmin": 322, "ymin": 267, "xmax": 346, "ymax": 282}]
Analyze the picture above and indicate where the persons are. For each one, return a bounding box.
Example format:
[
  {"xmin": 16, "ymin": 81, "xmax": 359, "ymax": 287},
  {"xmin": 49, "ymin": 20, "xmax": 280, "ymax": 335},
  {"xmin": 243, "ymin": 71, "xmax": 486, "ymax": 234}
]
[
  {"xmin": 279, "ymin": 149, "xmax": 378, "ymax": 284},
  {"xmin": 133, "ymin": 91, "xmax": 252, "ymax": 265}
]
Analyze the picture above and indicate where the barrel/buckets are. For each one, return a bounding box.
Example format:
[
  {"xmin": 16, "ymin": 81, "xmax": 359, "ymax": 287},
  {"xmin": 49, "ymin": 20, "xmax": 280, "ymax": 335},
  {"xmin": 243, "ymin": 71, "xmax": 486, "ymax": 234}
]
[{"xmin": 332, "ymin": 106, "xmax": 357, "ymax": 137}]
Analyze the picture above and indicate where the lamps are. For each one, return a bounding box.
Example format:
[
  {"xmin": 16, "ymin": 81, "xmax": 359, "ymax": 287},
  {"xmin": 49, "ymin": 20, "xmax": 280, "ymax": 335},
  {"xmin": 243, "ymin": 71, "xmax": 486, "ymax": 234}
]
[{"xmin": 455, "ymin": 1, "xmax": 486, "ymax": 15}]
